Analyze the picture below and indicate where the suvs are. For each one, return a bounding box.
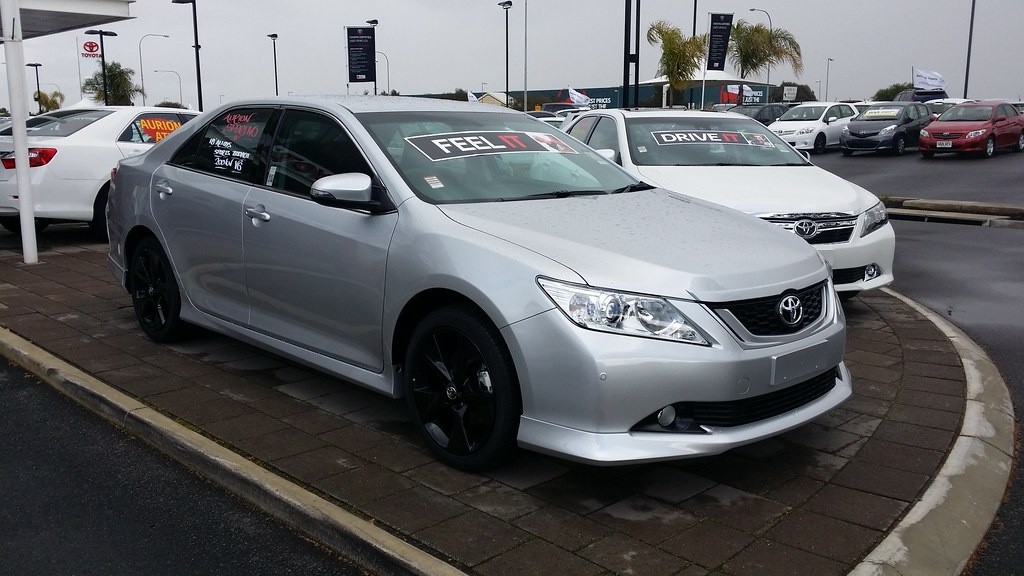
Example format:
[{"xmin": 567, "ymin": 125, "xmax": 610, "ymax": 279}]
[
  {"xmin": 726, "ymin": 102, "xmax": 788, "ymax": 126},
  {"xmin": 893, "ymin": 88, "xmax": 949, "ymax": 102}
]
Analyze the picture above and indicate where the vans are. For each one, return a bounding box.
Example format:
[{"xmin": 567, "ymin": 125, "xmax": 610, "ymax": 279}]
[{"xmin": 925, "ymin": 98, "xmax": 975, "ymax": 118}]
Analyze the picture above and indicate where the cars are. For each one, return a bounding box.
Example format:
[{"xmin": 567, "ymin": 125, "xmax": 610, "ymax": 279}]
[
  {"xmin": 839, "ymin": 101, "xmax": 932, "ymax": 156},
  {"xmin": 105, "ymin": 96, "xmax": 855, "ymax": 469},
  {"xmin": 561, "ymin": 110, "xmax": 896, "ymax": 303},
  {"xmin": 522, "ymin": 110, "xmax": 569, "ymax": 131},
  {"xmin": 919, "ymin": 101, "xmax": 1024, "ymax": 157},
  {"xmin": 768, "ymin": 101, "xmax": 860, "ymax": 154},
  {"xmin": 0, "ymin": 104, "xmax": 205, "ymax": 245}
]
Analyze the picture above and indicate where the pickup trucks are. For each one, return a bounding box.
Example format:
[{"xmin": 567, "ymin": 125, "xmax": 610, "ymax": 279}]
[{"xmin": 542, "ymin": 102, "xmax": 590, "ymax": 118}]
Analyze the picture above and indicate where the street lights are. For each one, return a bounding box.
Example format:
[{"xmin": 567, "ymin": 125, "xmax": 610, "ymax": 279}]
[
  {"xmin": 749, "ymin": 8, "xmax": 772, "ymax": 104},
  {"xmin": 155, "ymin": 70, "xmax": 182, "ymax": 105},
  {"xmin": 816, "ymin": 80, "xmax": 821, "ymax": 101},
  {"xmin": 498, "ymin": 0, "xmax": 512, "ymax": 107},
  {"xmin": 171, "ymin": 0, "xmax": 203, "ymax": 112},
  {"xmin": 139, "ymin": 34, "xmax": 169, "ymax": 106},
  {"xmin": 267, "ymin": 34, "xmax": 279, "ymax": 95},
  {"xmin": 84, "ymin": 29, "xmax": 117, "ymax": 107},
  {"xmin": 482, "ymin": 81, "xmax": 487, "ymax": 103},
  {"xmin": 825, "ymin": 58, "xmax": 833, "ymax": 101},
  {"xmin": 25, "ymin": 63, "xmax": 42, "ymax": 114}
]
